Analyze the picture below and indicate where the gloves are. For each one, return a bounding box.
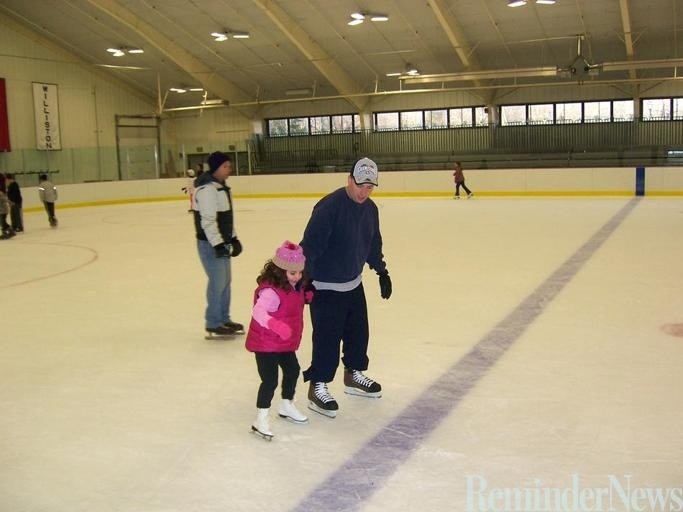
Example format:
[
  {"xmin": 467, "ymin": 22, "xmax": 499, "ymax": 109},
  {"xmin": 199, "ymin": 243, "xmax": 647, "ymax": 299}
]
[
  {"xmin": 214, "ymin": 243, "xmax": 230, "ymax": 259},
  {"xmin": 304, "ymin": 281, "xmax": 316, "ymax": 304},
  {"xmin": 376, "ymin": 270, "xmax": 392, "ymax": 300},
  {"xmin": 268, "ymin": 318, "xmax": 292, "ymax": 340},
  {"xmin": 181, "ymin": 188, "xmax": 187, "ymax": 194},
  {"xmin": 229, "ymin": 237, "xmax": 242, "ymax": 256}
]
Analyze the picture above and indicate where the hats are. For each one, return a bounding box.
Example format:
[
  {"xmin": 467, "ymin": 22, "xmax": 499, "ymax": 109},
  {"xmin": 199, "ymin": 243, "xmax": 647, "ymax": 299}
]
[
  {"xmin": 272, "ymin": 241, "xmax": 306, "ymax": 272},
  {"xmin": 187, "ymin": 169, "xmax": 195, "ymax": 177},
  {"xmin": 207, "ymin": 150, "xmax": 233, "ymax": 172},
  {"xmin": 352, "ymin": 156, "xmax": 379, "ymax": 187}
]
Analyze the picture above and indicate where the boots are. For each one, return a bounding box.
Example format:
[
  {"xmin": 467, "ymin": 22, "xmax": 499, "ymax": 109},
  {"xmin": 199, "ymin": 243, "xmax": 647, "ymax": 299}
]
[
  {"xmin": 251, "ymin": 407, "xmax": 274, "ymax": 437},
  {"xmin": 279, "ymin": 398, "xmax": 308, "ymax": 422},
  {"xmin": 0, "ymin": 223, "xmax": 24, "ymax": 240},
  {"xmin": 343, "ymin": 366, "xmax": 382, "ymax": 393},
  {"xmin": 307, "ymin": 377, "xmax": 339, "ymax": 410}
]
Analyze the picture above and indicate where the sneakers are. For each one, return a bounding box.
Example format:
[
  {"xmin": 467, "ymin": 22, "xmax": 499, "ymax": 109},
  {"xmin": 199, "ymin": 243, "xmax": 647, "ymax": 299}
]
[
  {"xmin": 49, "ymin": 216, "xmax": 58, "ymax": 226},
  {"xmin": 205, "ymin": 324, "xmax": 235, "ymax": 334},
  {"xmin": 453, "ymin": 196, "xmax": 460, "ymax": 198},
  {"xmin": 223, "ymin": 321, "xmax": 243, "ymax": 330},
  {"xmin": 188, "ymin": 209, "xmax": 194, "ymax": 212},
  {"xmin": 466, "ymin": 193, "xmax": 473, "ymax": 199}
]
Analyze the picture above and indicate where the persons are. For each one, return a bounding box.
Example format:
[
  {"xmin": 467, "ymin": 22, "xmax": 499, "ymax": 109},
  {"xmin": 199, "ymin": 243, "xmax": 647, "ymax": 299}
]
[
  {"xmin": 37, "ymin": 174, "xmax": 57, "ymax": 228},
  {"xmin": 0, "ymin": 173, "xmax": 14, "ymax": 240},
  {"xmin": 452, "ymin": 161, "xmax": 472, "ymax": 199},
  {"xmin": 243, "ymin": 240, "xmax": 316, "ymax": 439},
  {"xmin": 3, "ymin": 172, "xmax": 23, "ymax": 233},
  {"xmin": 295, "ymin": 156, "xmax": 392, "ymax": 413},
  {"xmin": 192, "ymin": 152, "xmax": 245, "ymax": 335},
  {"xmin": 181, "ymin": 169, "xmax": 196, "ymax": 213},
  {"xmin": 193, "ymin": 163, "xmax": 204, "ymax": 177}
]
